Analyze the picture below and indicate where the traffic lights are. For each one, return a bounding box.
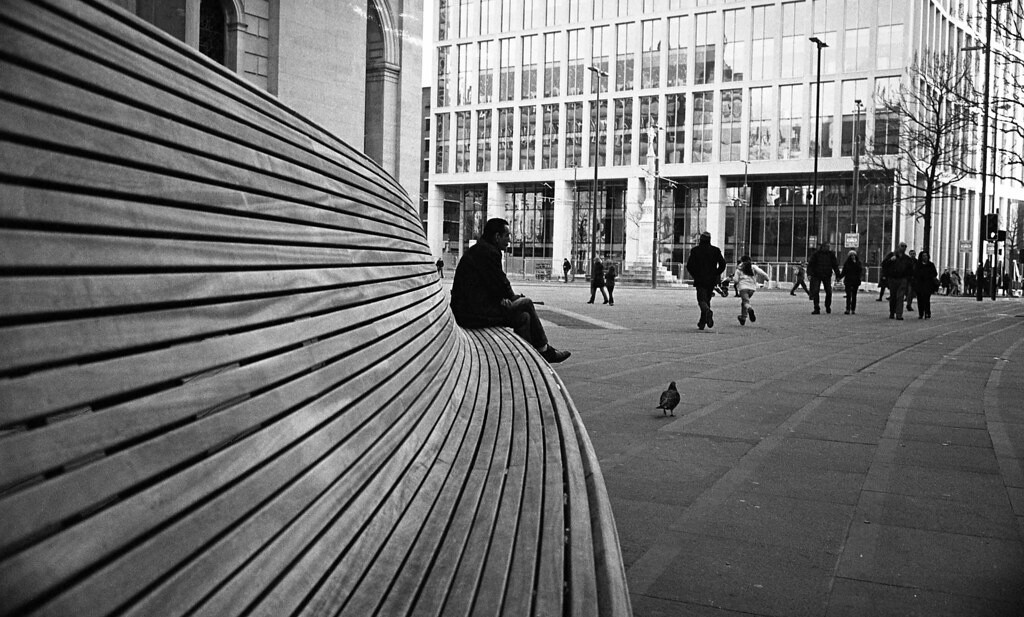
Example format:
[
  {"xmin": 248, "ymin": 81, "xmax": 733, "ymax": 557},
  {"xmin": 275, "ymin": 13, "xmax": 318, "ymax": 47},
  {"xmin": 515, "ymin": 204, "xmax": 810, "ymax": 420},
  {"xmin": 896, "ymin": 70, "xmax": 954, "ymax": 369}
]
[{"xmin": 984, "ymin": 214, "xmax": 999, "ymax": 242}]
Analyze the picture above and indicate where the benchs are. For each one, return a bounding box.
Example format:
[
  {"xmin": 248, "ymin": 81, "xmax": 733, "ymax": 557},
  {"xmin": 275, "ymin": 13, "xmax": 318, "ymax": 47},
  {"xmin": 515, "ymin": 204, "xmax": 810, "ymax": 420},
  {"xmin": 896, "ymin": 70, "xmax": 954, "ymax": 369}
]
[{"xmin": 0, "ymin": 0, "xmax": 632, "ymax": 617}]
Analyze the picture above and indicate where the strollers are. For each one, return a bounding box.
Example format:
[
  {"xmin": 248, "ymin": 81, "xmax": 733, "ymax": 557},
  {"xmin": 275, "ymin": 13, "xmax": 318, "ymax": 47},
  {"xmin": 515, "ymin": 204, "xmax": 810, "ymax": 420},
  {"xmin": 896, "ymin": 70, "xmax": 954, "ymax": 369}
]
[{"xmin": 711, "ymin": 274, "xmax": 735, "ymax": 297}]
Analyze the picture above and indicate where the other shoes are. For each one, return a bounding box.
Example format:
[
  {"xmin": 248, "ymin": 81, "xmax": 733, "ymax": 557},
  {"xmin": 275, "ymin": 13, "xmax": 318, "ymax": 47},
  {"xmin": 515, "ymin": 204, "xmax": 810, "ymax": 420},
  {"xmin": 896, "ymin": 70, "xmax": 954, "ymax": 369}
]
[
  {"xmin": 844, "ymin": 310, "xmax": 850, "ymax": 314},
  {"xmin": 918, "ymin": 314, "xmax": 931, "ymax": 320},
  {"xmin": 748, "ymin": 307, "xmax": 756, "ymax": 322},
  {"xmin": 826, "ymin": 308, "xmax": 831, "ymax": 314},
  {"xmin": 587, "ymin": 301, "xmax": 594, "ymax": 304},
  {"xmin": 876, "ymin": 298, "xmax": 882, "ymax": 301},
  {"xmin": 790, "ymin": 292, "xmax": 796, "ymax": 296},
  {"xmin": 851, "ymin": 310, "xmax": 856, "ymax": 314},
  {"xmin": 695, "ymin": 321, "xmax": 706, "ymax": 330},
  {"xmin": 906, "ymin": 304, "xmax": 914, "ymax": 311},
  {"xmin": 603, "ymin": 300, "xmax": 609, "ymax": 304},
  {"xmin": 811, "ymin": 309, "xmax": 821, "ymax": 315},
  {"xmin": 737, "ymin": 315, "xmax": 746, "ymax": 325},
  {"xmin": 706, "ymin": 310, "xmax": 713, "ymax": 328},
  {"xmin": 896, "ymin": 315, "xmax": 904, "ymax": 320},
  {"xmin": 889, "ymin": 314, "xmax": 895, "ymax": 319}
]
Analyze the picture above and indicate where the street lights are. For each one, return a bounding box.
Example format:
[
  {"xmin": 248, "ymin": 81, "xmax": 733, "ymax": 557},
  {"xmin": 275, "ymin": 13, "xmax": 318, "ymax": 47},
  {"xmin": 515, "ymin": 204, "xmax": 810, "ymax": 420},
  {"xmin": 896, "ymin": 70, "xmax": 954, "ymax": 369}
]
[
  {"xmin": 741, "ymin": 159, "xmax": 751, "ymax": 255},
  {"xmin": 589, "ymin": 67, "xmax": 608, "ymax": 280},
  {"xmin": 809, "ymin": 37, "xmax": 829, "ymax": 250},
  {"xmin": 652, "ymin": 125, "xmax": 664, "ymax": 288}
]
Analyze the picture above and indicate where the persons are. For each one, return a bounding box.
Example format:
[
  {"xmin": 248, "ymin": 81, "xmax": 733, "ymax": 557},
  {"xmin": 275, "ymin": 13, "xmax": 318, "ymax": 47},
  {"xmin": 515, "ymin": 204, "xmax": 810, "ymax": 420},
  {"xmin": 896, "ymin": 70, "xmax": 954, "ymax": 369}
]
[
  {"xmin": 436, "ymin": 258, "xmax": 444, "ymax": 278},
  {"xmin": 685, "ymin": 231, "xmax": 725, "ymax": 329},
  {"xmin": 965, "ymin": 261, "xmax": 1010, "ymax": 297},
  {"xmin": 450, "ymin": 219, "xmax": 571, "ymax": 364},
  {"xmin": 605, "ymin": 266, "xmax": 616, "ymax": 306},
  {"xmin": 734, "ymin": 255, "xmax": 769, "ymax": 326},
  {"xmin": 587, "ymin": 258, "xmax": 608, "ymax": 304},
  {"xmin": 564, "ymin": 258, "xmax": 569, "ymax": 283},
  {"xmin": 876, "ymin": 242, "xmax": 937, "ymax": 320},
  {"xmin": 940, "ymin": 268, "xmax": 961, "ymax": 296},
  {"xmin": 790, "ymin": 239, "xmax": 863, "ymax": 315}
]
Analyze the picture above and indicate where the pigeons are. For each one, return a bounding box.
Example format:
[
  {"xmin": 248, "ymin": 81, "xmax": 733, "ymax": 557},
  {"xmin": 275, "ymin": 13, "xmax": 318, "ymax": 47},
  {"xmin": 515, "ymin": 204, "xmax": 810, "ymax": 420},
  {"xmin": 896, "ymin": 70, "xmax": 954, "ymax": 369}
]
[{"xmin": 656, "ymin": 381, "xmax": 681, "ymax": 418}]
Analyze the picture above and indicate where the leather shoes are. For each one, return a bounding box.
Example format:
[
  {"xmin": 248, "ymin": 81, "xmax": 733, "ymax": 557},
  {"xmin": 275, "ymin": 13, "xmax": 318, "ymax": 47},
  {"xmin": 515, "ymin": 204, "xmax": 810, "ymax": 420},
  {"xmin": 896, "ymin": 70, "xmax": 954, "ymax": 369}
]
[{"xmin": 539, "ymin": 344, "xmax": 571, "ymax": 363}]
